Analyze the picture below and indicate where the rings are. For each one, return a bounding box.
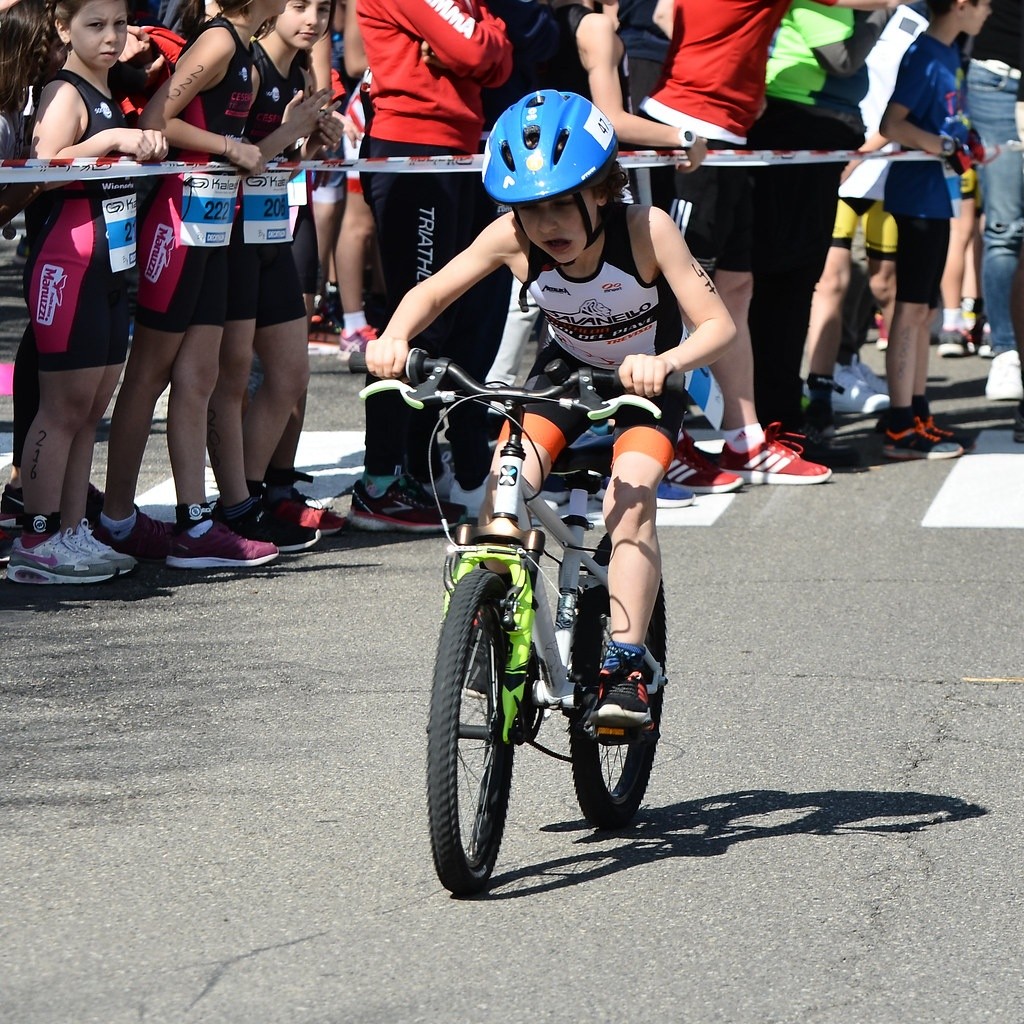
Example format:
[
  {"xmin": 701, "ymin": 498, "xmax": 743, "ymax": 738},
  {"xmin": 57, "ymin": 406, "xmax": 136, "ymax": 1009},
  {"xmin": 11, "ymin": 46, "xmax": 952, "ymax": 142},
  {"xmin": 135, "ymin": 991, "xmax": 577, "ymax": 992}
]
[{"xmin": 321, "ymin": 109, "xmax": 327, "ymax": 115}]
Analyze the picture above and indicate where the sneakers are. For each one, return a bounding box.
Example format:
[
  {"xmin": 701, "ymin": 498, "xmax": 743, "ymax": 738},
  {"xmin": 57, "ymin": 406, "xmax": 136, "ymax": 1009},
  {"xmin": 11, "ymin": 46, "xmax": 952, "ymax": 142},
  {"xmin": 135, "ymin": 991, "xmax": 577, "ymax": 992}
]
[
  {"xmin": 90, "ymin": 503, "xmax": 176, "ymax": 563},
  {"xmin": 937, "ymin": 320, "xmax": 995, "ymax": 359},
  {"xmin": 308, "ymin": 280, "xmax": 345, "ymax": 345},
  {"xmin": 595, "ymin": 476, "xmax": 697, "ymax": 508},
  {"xmin": 268, "ymin": 487, "xmax": 351, "ymax": 537},
  {"xmin": 338, "ymin": 324, "xmax": 384, "ymax": 353},
  {"xmin": 421, "ymin": 451, "xmax": 596, "ymax": 519},
  {"xmin": 212, "ymin": 496, "xmax": 322, "ymax": 553},
  {"xmin": 802, "ymin": 360, "xmax": 891, "ymax": 414},
  {"xmin": 67, "ymin": 517, "xmax": 140, "ymax": 577},
  {"xmin": 348, "ymin": 471, "xmax": 468, "ymax": 532},
  {"xmin": 589, "ymin": 643, "xmax": 652, "ymax": 729},
  {"xmin": 718, "ymin": 421, "xmax": 832, "ymax": 486},
  {"xmin": 0, "ymin": 482, "xmax": 140, "ymax": 567},
  {"xmin": 777, "ymin": 417, "xmax": 862, "ymax": 469},
  {"xmin": 461, "ymin": 610, "xmax": 490, "ymax": 699},
  {"xmin": 984, "ymin": 350, "xmax": 1024, "ymax": 401},
  {"xmin": 878, "ymin": 414, "xmax": 976, "ymax": 460},
  {"xmin": 165, "ymin": 519, "xmax": 280, "ymax": 570},
  {"xmin": 6, "ymin": 529, "xmax": 120, "ymax": 585},
  {"xmin": 663, "ymin": 428, "xmax": 745, "ymax": 495}
]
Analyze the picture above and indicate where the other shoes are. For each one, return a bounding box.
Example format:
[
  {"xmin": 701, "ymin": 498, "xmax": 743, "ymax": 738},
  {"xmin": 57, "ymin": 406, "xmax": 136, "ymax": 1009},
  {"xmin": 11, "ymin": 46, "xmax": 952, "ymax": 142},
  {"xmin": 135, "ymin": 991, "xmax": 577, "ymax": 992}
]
[{"xmin": 874, "ymin": 312, "xmax": 889, "ymax": 351}]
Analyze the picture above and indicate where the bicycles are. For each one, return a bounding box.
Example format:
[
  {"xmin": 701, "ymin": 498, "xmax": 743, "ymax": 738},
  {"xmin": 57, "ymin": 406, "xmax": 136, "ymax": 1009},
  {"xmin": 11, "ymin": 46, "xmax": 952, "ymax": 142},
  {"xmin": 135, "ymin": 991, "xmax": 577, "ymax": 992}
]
[{"xmin": 348, "ymin": 347, "xmax": 668, "ymax": 894}]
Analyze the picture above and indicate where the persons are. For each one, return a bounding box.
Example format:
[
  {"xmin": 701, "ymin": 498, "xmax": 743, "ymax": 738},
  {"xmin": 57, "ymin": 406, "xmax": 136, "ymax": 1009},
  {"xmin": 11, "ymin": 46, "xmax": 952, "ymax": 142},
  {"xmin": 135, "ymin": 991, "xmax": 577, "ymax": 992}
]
[
  {"xmin": 368, "ymin": 88, "xmax": 736, "ymax": 722},
  {"xmin": 336, "ymin": 1, "xmax": 1024, "ymax": 530},
  {"xmin": 0, "ymin": 0, "xmax": 349, "ymax": 583}
]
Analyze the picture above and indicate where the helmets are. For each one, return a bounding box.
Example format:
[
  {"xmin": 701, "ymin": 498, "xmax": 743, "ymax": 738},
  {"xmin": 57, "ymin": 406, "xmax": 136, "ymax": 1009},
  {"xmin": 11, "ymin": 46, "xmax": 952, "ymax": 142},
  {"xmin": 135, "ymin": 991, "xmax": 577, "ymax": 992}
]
[{"xmin": 482, "ymin": 88, "xmax": 619, "ymax": 207}]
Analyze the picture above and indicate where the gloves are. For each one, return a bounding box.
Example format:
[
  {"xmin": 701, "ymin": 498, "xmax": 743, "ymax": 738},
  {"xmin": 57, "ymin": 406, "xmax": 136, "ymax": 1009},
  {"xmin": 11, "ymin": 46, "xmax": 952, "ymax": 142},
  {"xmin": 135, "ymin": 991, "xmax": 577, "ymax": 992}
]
[
  {"xmin": 948, "ymin": 145, "xmax": 973, "ymax": 175},
  {"xmin": 967, "ymin": 129, "xmax": 986, "ymax": 161}
]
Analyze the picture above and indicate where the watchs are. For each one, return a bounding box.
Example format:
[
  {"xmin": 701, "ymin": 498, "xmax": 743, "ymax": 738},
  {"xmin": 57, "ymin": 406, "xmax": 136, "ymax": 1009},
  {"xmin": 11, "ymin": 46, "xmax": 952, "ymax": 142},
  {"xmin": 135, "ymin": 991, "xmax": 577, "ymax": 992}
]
[
  {"xmin": 942, "ymin": 137, "xmax": 953, "ymax": 153},
  {"xmin": 679, "ymin": 127, "xmax": 696, "ymax": 145}
]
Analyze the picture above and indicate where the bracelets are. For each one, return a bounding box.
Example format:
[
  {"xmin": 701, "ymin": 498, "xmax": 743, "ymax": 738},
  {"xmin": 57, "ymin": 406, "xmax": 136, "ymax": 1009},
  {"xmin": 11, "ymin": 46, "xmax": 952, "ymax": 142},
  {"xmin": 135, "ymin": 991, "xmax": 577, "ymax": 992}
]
[{"xmin": 222, "ymin": 135, "xmax": 227, "ymax": 155}]
